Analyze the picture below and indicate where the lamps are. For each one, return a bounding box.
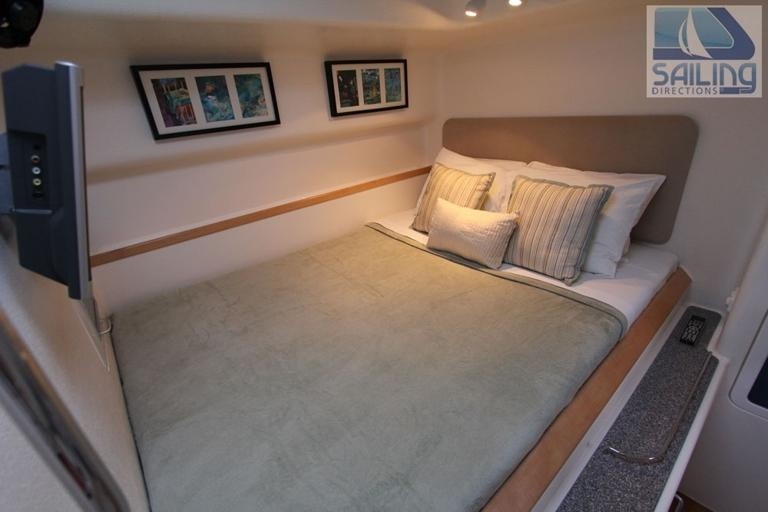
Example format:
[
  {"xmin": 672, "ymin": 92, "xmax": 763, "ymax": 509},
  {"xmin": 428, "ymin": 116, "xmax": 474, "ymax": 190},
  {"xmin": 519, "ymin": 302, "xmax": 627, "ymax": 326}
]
[{"xmin": 465, "ymin": 0, "xmax": 487, "ymax": 17}]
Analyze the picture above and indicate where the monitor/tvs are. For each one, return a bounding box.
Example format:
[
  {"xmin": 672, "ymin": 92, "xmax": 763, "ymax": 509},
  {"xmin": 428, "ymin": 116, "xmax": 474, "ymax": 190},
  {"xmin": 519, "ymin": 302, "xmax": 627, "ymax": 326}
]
[{"xmin": 0, "ymin": 60, "xmax": 95, "ymax": 300}]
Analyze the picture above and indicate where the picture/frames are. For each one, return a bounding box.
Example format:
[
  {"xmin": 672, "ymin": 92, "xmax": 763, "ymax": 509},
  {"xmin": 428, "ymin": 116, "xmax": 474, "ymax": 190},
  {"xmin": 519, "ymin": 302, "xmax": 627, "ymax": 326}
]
[
  {"xmin": 129, "ymin": 61, "xmax": 281, "ymax": 139},
  {"xmin": 324, "ymin": 59, "xmax": 409, "ymax": 117}
]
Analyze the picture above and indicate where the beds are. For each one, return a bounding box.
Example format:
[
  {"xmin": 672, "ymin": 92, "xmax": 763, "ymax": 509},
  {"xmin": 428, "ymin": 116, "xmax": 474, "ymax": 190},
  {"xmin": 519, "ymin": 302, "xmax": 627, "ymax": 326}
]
[{"xmin": 111, "ymin": 116, "xmax": 727, "ymax": 512}]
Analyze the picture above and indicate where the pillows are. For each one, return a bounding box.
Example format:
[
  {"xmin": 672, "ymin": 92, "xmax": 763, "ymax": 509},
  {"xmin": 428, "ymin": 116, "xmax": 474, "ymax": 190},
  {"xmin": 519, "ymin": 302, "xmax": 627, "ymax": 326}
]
[
  {"xmin": 411, "ymin": 163, "xmax": 496, "ymax": 235},
  {"xmin": 529, "ymin": 161, "xmax": 667, "ymax": 255},
  {"xmin": 520, "ymin": 168, "xmax": 659, "ymax": 276},
  {"xmin": 413, "ymin": 148, "xmax": 520, "ymax": 213},
  {"xmin": 426, "ymin": 198, "xmax": 519, "ymax": 270},
  {"xmin": 477, "ymin": 157, "xmax": 528, "ymax": 171},
  {"xmin": 501, "ymin": 175, "xmax": 615, "ymax": 284}
]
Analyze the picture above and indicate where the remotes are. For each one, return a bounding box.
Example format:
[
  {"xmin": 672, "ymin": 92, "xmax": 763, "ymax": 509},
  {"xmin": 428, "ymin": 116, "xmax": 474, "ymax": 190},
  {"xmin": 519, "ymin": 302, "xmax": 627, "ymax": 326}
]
[{"xmin": 679, "ymin": 313, "xmax": 707, "ymax": 344}]
